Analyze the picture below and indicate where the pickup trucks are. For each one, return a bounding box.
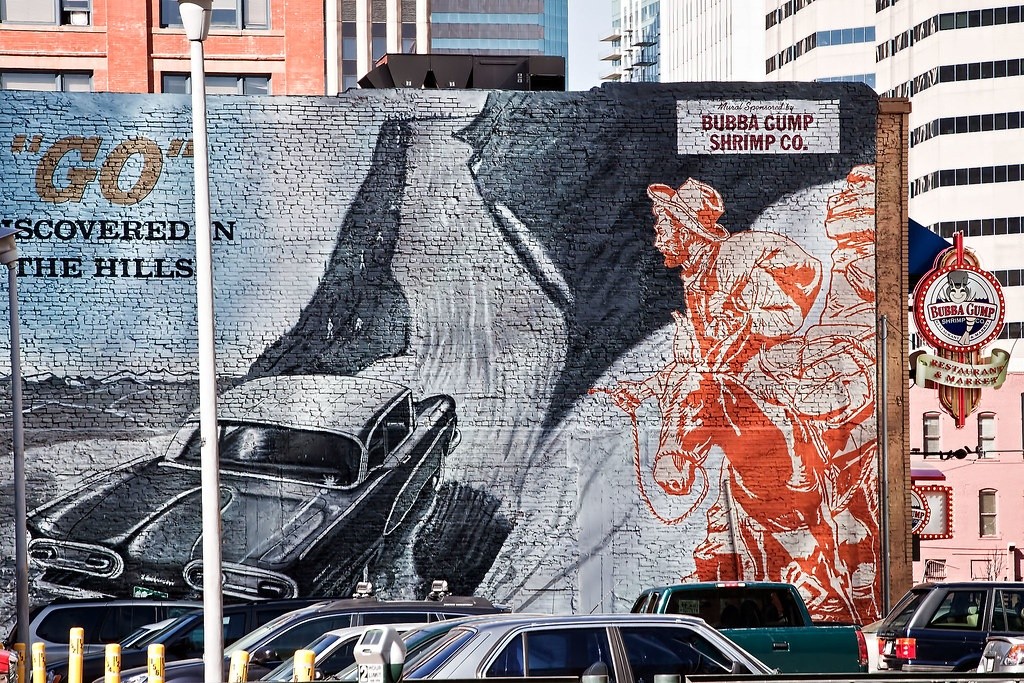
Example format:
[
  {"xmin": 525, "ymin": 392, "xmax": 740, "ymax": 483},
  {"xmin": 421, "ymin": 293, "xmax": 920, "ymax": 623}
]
[{"xmin": 630, "ymin": 581, "xmax": 869, "ymax": 678}]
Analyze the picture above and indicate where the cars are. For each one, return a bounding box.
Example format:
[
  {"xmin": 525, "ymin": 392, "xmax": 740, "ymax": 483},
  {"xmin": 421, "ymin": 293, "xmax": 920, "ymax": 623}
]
[
  {"xmin": 256, "ymin": 611, "xmax": 777, "ymax": 683},
  {"xmin": 26, "ymin": 374, "xmax": 462, "ymax": 600}
]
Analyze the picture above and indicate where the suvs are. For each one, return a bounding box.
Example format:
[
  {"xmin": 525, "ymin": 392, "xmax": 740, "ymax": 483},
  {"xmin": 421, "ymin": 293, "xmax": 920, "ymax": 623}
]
[
  {"xmin": 92, "ymin": 581, "xmax": 507, "ymax": 682},
  {"xmin": 0, "ymin": 596, "xmax": 205, "ymax": 683},
  {"xmin": 35, "ymin": 593, "xmax": 333, "ymax": 683},
  {"xmin": 874, "ymin": 578, "xmax": 1023, "ymax": 678}
]
